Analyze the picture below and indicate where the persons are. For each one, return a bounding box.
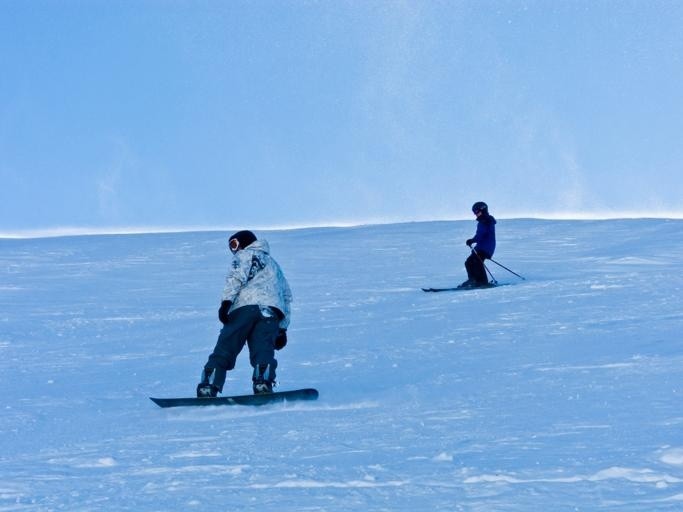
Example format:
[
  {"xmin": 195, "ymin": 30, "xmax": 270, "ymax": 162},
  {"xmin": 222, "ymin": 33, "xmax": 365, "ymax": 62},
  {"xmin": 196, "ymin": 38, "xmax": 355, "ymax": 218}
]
[
  {"xmin": 196, "ymin": 229, "xmax": 293, "ymax": 397},
  {"xmin": 456, "ymin": 201, "xmax": 496, "ymax": 288}
]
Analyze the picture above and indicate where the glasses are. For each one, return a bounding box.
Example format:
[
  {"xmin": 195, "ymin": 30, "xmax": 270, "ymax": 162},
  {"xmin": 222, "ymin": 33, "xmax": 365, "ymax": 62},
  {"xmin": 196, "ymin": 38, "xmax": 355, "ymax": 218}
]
[{"xmin": 229, "ymin": 238, "xmax": 239, "ymax": 255}]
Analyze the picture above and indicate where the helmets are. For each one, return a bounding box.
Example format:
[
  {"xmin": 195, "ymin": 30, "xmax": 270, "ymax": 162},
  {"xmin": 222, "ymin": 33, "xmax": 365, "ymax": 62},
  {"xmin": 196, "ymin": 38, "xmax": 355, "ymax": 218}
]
[{"xmin": 472, "ymin": 201, "xmax": 488, "ymax": 215}]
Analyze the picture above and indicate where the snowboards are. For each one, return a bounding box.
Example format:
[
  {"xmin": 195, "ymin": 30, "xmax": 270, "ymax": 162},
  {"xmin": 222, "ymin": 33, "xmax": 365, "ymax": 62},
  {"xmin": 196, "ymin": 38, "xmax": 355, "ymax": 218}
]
[
  {"xmin": 420, "ymin": 281, "xmax": 514, "ymax": 292},
  {"xmin": 150, "ymin": 388, "xmax": 320, "ymax": 408}
]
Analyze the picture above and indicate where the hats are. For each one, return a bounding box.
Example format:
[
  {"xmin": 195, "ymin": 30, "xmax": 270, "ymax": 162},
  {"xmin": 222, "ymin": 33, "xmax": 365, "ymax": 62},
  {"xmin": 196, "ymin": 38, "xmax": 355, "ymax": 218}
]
[{"xmin": 229, "ymin": 229, "xmax": 257, "ymax": 250}]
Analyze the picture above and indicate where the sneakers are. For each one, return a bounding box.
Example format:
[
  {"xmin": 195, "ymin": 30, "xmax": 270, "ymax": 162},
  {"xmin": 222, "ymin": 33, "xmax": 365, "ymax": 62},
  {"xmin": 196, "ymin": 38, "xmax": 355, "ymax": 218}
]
[
  {"xmin": 196, "ymin": 382, "xmax": 218, "ymax": 397},
  {"xmin": 253, "ymin": 382, "xmax": 275, "ymax": 394}
]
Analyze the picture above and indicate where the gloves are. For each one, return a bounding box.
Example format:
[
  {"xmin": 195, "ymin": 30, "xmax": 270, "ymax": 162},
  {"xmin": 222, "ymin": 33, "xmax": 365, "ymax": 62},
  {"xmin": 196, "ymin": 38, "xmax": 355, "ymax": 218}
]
[
  {"xmin": 466, "ymin": 238, "xmax": 474, "ymax": 247},
  {"xmin": 274, "ymin": 328, "xmax": 287, "ymax": 351},
  {"xmin": 217, "ymin": 300, "xmax": 232, "ymax": 323}
]
